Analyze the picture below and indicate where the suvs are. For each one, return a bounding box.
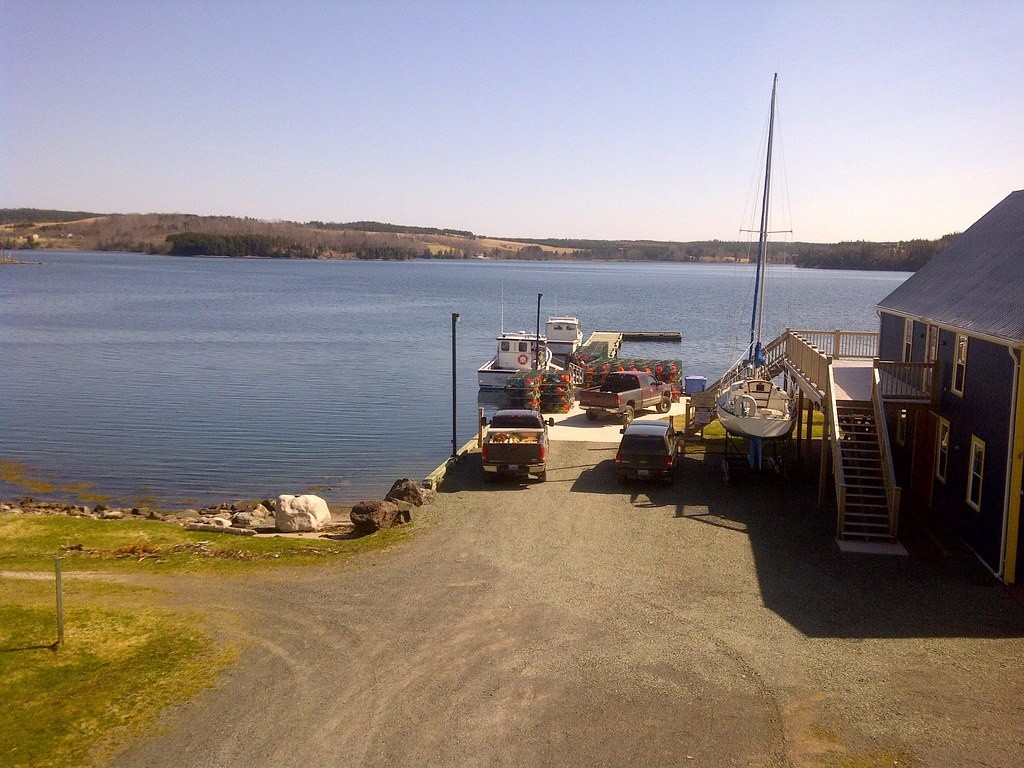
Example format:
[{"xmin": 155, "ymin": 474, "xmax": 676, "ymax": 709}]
[{"xmin": 616, "ymin": 419, "xmax": 682, "ymax": 484}]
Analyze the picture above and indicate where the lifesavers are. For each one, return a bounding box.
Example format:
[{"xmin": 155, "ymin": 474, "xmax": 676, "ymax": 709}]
[
  {"xmin": 735, "ymin": 395, "xmax": 757, "ymax": 417},
  {"xmin": 518, "ymin": 355, "xmax": 528, "ymax": 364}
]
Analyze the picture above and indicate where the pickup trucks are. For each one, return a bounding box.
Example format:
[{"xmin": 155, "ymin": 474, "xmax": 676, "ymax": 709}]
[
  {"xmin": 578, "ymin": 371, "xmax": 672, "ymax": 424},
  {"xmin": 481, "ymin": 409, "xmax": 554, "ymax": 482}
]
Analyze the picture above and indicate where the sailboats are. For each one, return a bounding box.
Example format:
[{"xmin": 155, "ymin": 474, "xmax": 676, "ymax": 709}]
[{"xmin": 710, "ymin": 71, "xmax": 798, "ymax": 440}]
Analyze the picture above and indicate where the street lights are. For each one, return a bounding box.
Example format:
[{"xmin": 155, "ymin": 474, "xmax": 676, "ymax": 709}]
[{"xmin": 451, "ymin": 313, "xmax": 461, "ymax": 457}]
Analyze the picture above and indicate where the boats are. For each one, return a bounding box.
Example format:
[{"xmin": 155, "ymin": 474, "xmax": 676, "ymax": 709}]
[
  {"xmin": 542, "ymin": 295, "xmax": 584, "ymax": 354},
  {"xmin": 477, "ymin": 279, "xmax": 552, "ymax": 390}
]
